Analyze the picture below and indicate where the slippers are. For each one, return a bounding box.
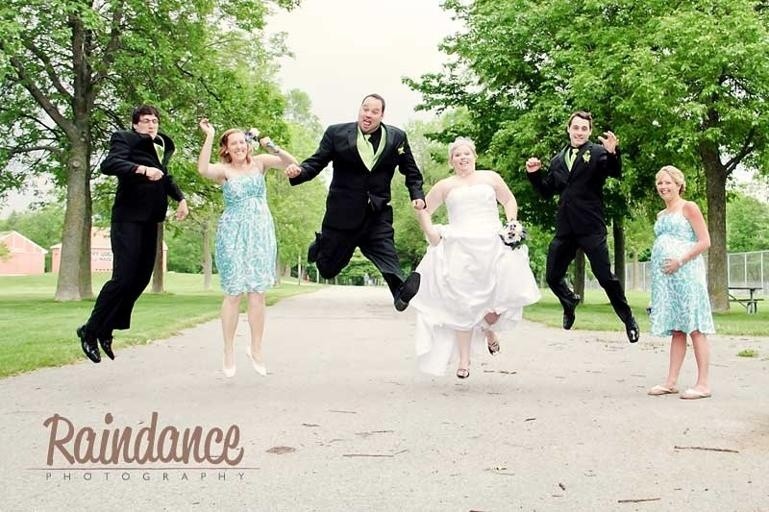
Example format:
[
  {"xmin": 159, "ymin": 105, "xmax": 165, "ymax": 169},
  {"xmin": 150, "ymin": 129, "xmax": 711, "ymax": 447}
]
[
  {"xmin": 649, "ymin": 383, "xmax": 677, "ymax": 397},
  {"xmin": 679, "ymin": 385, "xmax": 714, "ymax": 402}
]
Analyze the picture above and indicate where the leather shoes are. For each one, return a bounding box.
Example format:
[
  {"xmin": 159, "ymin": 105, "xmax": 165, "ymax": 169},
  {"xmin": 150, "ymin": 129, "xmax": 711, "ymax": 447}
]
[
  {"xmin": 562, "ymin": 294, "xmax": 581, "ymax": 330},
  {"xmin": 393, "ymin": 272, "xmax": 420, "ymax": 312},
  {"xmin": 625, "ymin": 318, "xmax": 640, "ymax": 343},
  {"xmin": 77, "ymin": 325, "xmax": 101, "ymax": 363},
  {"xmin": 97, "ymin": 331, "xmax": 115, "ymax": 360}
]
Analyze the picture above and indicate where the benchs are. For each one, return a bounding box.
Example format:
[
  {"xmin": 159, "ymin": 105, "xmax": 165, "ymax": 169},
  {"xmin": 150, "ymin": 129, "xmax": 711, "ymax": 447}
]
[{"xmin": 728, "ymin": 299, "xmax": 764, "ymax": 313}]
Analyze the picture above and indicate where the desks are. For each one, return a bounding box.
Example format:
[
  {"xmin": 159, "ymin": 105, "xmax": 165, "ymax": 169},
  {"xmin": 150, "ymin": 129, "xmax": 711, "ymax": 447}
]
[{"xmin": 727, "ymin": 286, "xmax": 763, "ymax": 314}]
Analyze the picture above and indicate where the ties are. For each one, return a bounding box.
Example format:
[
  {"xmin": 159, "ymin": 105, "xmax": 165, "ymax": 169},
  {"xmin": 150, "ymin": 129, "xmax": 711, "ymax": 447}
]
[
  {"xmin": 570, "ymin": 148, "xmax": 579, "ymax": 166},
  {"xmin": 364, "ymin": 134, "xmax": 375, "ymax": 157}
]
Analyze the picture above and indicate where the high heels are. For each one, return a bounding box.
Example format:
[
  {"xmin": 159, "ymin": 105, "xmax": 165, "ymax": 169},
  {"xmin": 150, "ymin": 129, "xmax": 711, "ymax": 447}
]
[
  {"xmin": 224, "ymin": 350, "xmax": 237, "ymax": 377},
  {"xmin": 246, "ymin": 346, "xmax": 268, "ymax": 378}
]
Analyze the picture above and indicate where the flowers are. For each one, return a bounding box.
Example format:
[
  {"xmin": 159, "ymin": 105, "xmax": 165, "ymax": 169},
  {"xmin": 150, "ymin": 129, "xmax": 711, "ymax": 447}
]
[
  {"xmin": 244, "ymin": 127, "xmax": 274, "ymax": 150},
  {"xmin": 500, "ymin": 219, "xmax": 529, "ymax": 251}
]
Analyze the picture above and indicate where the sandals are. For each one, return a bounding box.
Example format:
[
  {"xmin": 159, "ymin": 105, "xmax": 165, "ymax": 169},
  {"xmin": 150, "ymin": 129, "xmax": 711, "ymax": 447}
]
[
  {"xmin": 486, "ymin": 337, "xmax": 501, "ymax": 355},
  {"xmin": 456, "ymin": 358, "xmax": 471, "ymax": 380}
]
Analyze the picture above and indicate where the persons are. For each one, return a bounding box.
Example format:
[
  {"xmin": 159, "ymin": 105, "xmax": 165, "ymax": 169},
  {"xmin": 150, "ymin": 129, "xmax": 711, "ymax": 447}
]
[
  {"xmin": 647, "ymin": 165, "xmax": 717, "ymax": 399},
  {"xmin": 284, "ymin": 94, "xmax": 427, "ymax": 311},
  {"xmin": 409, "ymin": 136, "xmax": 541, "ymax": 379},
  {"xmin": 525, "ymin": 110, "xmax": 640, "ymax": 343},
  {"xmin": 77, "ymin": 104, "xmax": 190, "ymax": 363},
  {"xmin": 197, "ymin": 118, "xmax": 299, "ymax": 378}
]
